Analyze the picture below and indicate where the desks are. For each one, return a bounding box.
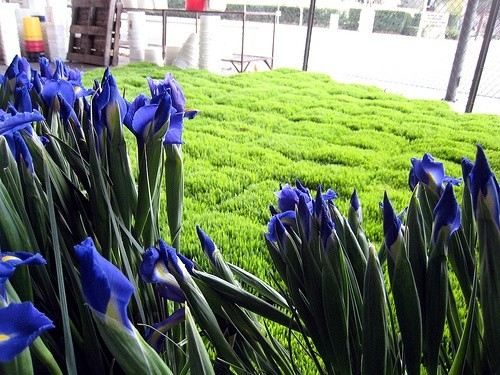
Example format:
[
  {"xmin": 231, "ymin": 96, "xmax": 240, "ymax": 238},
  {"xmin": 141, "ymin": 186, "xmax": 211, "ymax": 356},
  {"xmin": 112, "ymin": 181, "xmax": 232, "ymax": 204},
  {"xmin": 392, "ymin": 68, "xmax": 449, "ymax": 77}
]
[{"xmin": 122, "ymin": 7, "xmax": 277, "ymax": 73}]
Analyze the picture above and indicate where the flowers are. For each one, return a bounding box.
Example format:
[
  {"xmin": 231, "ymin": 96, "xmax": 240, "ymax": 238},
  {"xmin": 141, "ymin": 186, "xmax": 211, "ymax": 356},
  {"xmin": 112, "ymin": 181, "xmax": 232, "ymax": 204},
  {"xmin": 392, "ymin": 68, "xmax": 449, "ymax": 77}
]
[{"xmin": 1, "ymin": 53, "xmax": 500, "ymax": 374}]
